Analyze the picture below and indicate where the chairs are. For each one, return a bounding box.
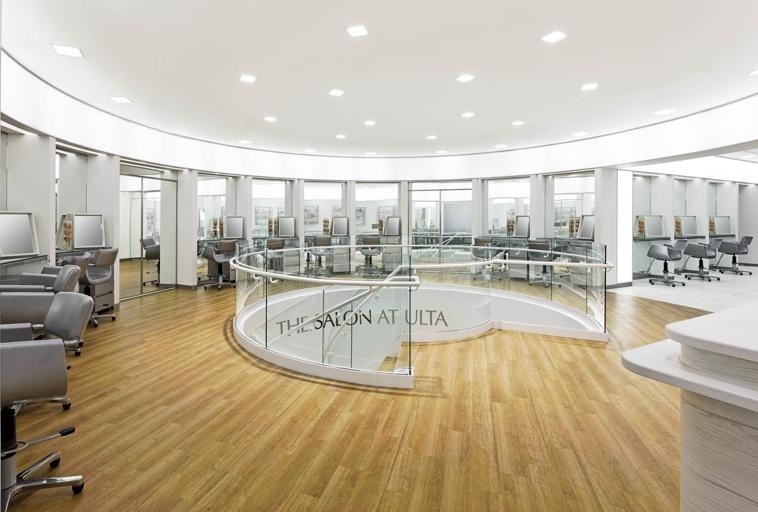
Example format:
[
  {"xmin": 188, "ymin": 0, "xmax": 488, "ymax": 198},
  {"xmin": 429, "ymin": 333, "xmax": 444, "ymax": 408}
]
[
  {"xmin": 202, "ymin": 237, "xmax": 385, "ymax": 291},
  {"xmin": 1, "ymin": 248, "xmax": 118, "ymax": 512},
  {"xmin": 469, "ymin": 241, "xmax": 569, "ymax": 288},
  {"xmin": 633, "ymin": 236, "xmax": 753, "ymax": 287},
  {"xmin": 140, "ymin": 237, "xmax": 159, "ymax": 286}
]
